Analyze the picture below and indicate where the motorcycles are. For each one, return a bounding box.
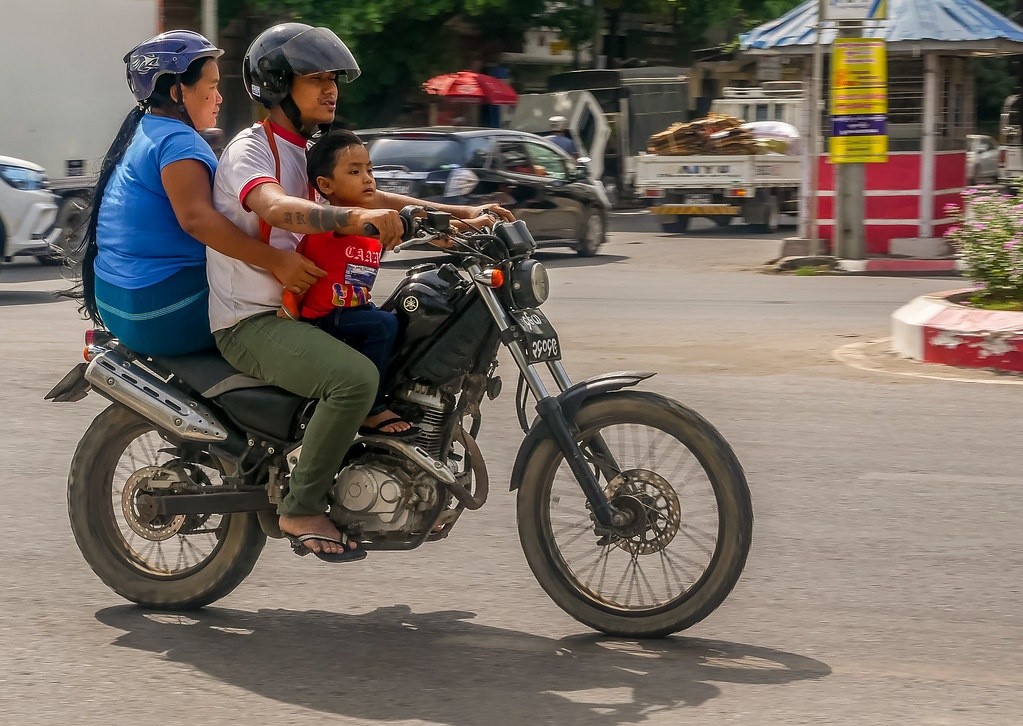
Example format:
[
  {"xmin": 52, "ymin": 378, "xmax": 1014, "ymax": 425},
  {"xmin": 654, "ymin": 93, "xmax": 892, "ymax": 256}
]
[{"xmin": 43, "ymin": 205, "xmax": 755, "ymax": 642}]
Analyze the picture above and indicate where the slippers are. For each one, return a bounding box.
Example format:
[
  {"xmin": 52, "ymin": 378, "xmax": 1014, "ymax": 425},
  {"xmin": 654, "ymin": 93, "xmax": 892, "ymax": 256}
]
[
  {"xmin": 285, "ymin": 513, "xmax": 368, "ymax": 564},
  {"xmin": 357, "ymin": 408, "xmax": 422, "ymax": 437}
]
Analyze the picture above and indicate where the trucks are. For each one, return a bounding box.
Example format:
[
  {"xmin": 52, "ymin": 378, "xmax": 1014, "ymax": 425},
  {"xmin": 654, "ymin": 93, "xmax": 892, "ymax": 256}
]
[{"xmin": 624, "ymin": 81, "xmax": 826, "ymax": 231}]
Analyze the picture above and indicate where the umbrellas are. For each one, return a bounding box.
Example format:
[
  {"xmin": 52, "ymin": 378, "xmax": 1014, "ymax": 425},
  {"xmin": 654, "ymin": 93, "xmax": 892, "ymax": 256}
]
[{"xmin": 421, "ymin": 70, "xmax": 518, "ymax": 105}]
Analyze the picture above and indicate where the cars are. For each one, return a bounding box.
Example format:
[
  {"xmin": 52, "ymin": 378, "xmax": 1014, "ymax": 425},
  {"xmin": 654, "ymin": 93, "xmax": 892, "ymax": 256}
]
[
  {"xmin": 350, "ymin": 125, "xmax": 609, "ymax": 258},
  {"xmin": 0, "ymin": 156, "xmax": 72, "ymax": 269},
  {"xmin": 994, "ymin": 93, "xmax": 1023, "ymax": 199},
  {"xmin": 966, "ymin": 133, "xmax": 999, "ymax": 186}
]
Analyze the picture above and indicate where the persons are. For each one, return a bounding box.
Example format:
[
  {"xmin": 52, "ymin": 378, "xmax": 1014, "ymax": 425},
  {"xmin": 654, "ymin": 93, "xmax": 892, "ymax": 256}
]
[
  {"xmin": 545, "ymin": 116, "xmax": 579, "ymax": 161},
  {"xmin": 46, "ymin": 30, "xmax": 327, "ymax": 356},
  {"xmin": 206, "ymin": 23, "xmax": 517, "ymax": 563}
]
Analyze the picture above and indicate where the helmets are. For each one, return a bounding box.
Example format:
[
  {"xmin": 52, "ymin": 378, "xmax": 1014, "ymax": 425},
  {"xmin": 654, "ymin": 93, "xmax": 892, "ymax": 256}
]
[
  {"xmin": 242, "ymin": 23, "xmax": 346, "ymax": 108},
  {"xmin": 122, "ymin": 29, "xmax": 225, "ymax": 101}
]
